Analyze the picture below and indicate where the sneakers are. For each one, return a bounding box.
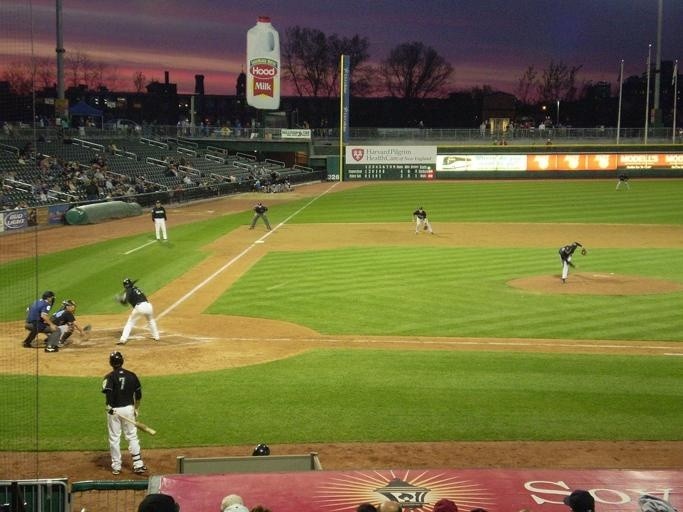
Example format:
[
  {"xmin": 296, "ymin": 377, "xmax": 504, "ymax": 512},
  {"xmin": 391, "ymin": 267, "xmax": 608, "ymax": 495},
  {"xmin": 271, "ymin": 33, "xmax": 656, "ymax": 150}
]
[{"xmin": 45, "ymin": 343, "xmax": 64, "ymax": 352}]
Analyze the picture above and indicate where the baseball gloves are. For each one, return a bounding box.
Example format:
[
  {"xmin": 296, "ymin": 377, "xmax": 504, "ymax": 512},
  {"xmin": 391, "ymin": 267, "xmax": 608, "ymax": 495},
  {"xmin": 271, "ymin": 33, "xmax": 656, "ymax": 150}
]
[
  {"xmin": 424, "ymin": 225, "xmax": 427, "ymax": 230},
  {"xmin": 581, "ymin": 248, "xmax": 587, "ymax": 255},
  {"xmin": 83, "ymin": 325, "xmax": 92, "ymax": 332}
]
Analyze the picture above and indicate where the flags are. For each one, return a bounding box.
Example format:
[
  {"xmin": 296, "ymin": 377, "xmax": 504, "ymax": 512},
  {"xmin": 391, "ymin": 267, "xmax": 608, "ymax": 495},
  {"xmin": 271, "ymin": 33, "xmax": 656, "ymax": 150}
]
[{"xmin": 614, "ymin": 55, "xmax": 677, "ymax": 99}]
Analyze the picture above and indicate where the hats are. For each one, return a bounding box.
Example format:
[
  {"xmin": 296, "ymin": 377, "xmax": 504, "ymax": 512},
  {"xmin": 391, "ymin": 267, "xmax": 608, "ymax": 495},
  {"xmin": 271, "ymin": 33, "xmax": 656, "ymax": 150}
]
[
  {"xmin": 563, "ymin": 490, "xmax": 594, "ymax": 509},
  {"xmin": 220, "ymin": 494, "xmax": 250, "ymax": 512}
]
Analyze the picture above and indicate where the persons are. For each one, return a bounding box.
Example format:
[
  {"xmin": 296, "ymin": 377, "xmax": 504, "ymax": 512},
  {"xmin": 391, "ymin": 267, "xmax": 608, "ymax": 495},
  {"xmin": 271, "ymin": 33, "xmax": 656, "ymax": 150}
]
[
  {"xmin": 136, "ymin": 491, "xmax": 676, "ymax": 512},
  {"xmin": 99, "ymin": 350, "xmax": 148, "ymax": 476},
  {"xmin": 250, "ymin": 442, "xmax": 271, "ymax": 456},
  {"xmin": 247, "ymin": 202, "xmax": 272, "ymax": 231},
  {"xmin": 296, "ymin": 116, "xmax": 559, "ymax": 148},
  {"xmin": 21, "ymin": 290, "xmax": 62, "ymax": 353},
  {"xmin": 410, "ymin": 207, "xmax": 435, "ymax": 236},
  {"xmin": 43, "ymin": 299, "xmax": 85, "ymax": 347},
  {"xmin": 150, "ymin": 200, "xmax": 168, "ymax": 241},
  {"xmin": 112, "ymin": 278, "xmax": 160, "ymax": 345},
  {"xmin": 615, "ymin": 173, "xmax": 633, "ymax": 192},
  {"xmin": 558, "ymin": 241, "xmax": 587, "ymax": 285},
  {"xmin": 0, "ymin": 112, "xmax": 292, "ymax": 201}
]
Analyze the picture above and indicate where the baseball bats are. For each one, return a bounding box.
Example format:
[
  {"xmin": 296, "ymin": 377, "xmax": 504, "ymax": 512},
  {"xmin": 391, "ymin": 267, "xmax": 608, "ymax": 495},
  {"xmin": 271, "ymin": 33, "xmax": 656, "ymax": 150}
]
[
  {"xmin": 113, "ymin": 411, "xmax": 156, "ymax": 436},
  {"xmin": 119, "ymin": 279, "xmax": 138, "ymax": 295}
]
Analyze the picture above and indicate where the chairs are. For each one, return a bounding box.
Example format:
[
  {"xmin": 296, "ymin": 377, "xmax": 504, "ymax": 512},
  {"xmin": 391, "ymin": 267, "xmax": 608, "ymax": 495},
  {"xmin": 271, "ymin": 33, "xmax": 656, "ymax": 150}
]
[{"xmin": 0, "ymin": 122, "xmax": 317, "ymax": 219}]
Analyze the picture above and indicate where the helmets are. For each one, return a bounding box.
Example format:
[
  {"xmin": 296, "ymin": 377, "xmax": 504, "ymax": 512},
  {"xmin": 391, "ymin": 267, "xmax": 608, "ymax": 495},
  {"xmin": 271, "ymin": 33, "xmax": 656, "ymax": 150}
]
[
  {"xmin": 62, "ymin": 299, "xmax": 76, "ymax": 313},
  {"xmin": 253, "ymin": 443, "xmax": 270, "ymax": 456},
  {"xmin": 123, "ymin": 279, "xmax": 132, "ymax": 288},
  {"xmin": 110, "ymin": 351, "xmax": 123, "ymax": 367}
]
